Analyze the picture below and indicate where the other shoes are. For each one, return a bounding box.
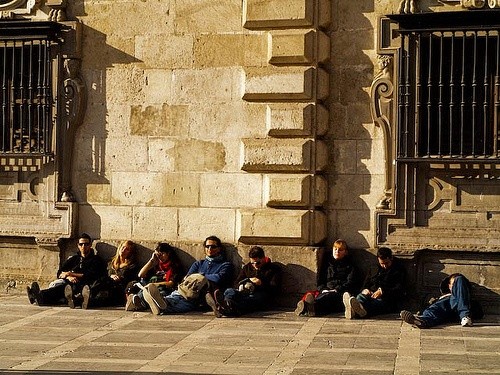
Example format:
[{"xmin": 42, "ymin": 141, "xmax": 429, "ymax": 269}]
[
  {"xmin": 82, "ymin": 284, "xmax": 92, "ymax": 310},
  {"xmin": 206, "ymin": 292, "xmax": 223, "ymax": 318},
  {"xmin": 126, "ymin": 293, "xmax": 146, "ymax": 311},
  {"xmin": 142, "ymin": 289, "xmax": 161, "ymax": 315},
  {"xmin": 351, "ymin": 296, "xmax": 368, "ymax": 318},
  {"xmin": 27, "ymin": 282, "xmax": 42, "ymax": 306},
  {"xmin": 64, "ymin": 285, "xmax": 78, "ymax": 308},
  {"xmin": 214, "ymin": 290, "xmax": 230, "ymax": 311},
  {"xmin": 342, "ymin": 292, "xmax": 356, "ymax": 319},
  {"xmin": 295, "ymin": 300, "xmax": 307, "ymax": 316},
  {"xmin": 148, "ymin": 283, "xmax": 167, "ymax": 310},
  {"xmin": 306, "ymin": 293, "xmax": 316, "ymax": 317}
]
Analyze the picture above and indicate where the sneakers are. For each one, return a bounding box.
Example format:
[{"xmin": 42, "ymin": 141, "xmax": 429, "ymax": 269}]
[
  {"xmin": 461, "ymin": 317, "xmax": 472, "ymax": 327},
  {"xmin": 400, "ymin": 310, "xmax": 424, "ymax": 328}
]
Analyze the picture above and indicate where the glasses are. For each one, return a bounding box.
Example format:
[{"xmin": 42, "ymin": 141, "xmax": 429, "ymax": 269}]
[
  {"xmin": 78, "ymin": 242, "xmax": 91, "ymax": 247},
  {"xmin": 205, "ymin": 244, "xmax": 222, "ymax": 248},
  {"xmin": 250, "ymin": 257, "xmax": 261, "ymax": 265}
]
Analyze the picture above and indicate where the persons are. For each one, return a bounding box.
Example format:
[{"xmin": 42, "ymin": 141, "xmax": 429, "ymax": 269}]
[
  {"xmin": 294, "ymin": 238, "xmax": 364, "ymax": 317},
  {"xmin": 343, "ymin": 247, "xmax": 409, "ymax": 319},
  {"xmin": 400, "ymin": 274, "xmax": 477, "ymax": 328},
  {"xmin": 27, "ymin": 233, "xmax": 283, "ymax": 318}
]
